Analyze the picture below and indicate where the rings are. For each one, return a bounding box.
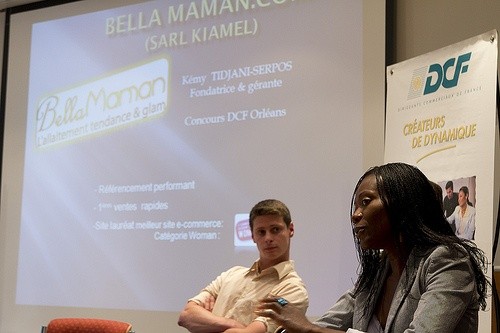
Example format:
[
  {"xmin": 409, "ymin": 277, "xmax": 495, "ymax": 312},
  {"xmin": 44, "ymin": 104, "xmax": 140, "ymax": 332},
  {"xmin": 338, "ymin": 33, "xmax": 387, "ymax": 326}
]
[{"xmin": 275, "ymin": 297, "xmax": 288, "ymax": 306}]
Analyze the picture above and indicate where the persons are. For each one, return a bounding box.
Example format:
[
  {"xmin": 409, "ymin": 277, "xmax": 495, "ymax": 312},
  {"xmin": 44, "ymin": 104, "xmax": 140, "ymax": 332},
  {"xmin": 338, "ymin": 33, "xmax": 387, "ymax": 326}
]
[
  {"xmin": 254, "ymin": 162, "xmax": 493, "ymax": 333},
  {"xmin": 178, "ymin": 199, "xmax": 309, "ymax": 333},
  {"xmin": 446, "ymin": 186, "xmax": 476, "ymax": 241},
  {"xmin": 442, "ymin": 181, "xmax": 473, "ymax": 235}
]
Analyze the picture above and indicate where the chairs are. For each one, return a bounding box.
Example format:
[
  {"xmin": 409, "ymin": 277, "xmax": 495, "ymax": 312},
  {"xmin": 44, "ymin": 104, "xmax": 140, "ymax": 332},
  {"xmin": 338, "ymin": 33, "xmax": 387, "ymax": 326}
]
[{"xmin": 41, "ymin": 318, "xmax": 134, "ymax": 333}]
